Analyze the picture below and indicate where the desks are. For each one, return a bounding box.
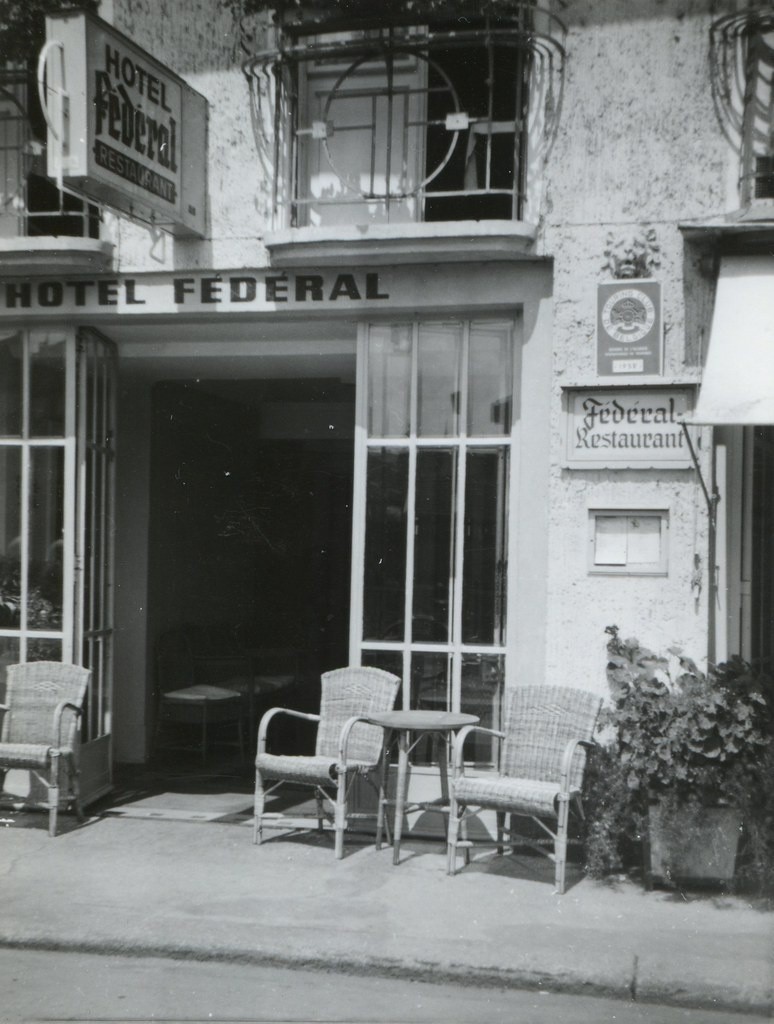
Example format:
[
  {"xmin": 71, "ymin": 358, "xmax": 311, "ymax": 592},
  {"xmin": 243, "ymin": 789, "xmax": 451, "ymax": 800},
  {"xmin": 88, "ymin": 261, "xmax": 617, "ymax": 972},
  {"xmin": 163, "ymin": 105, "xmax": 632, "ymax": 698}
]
[{"xmin": 366, "ymin": 709, "xmax": 481, "ymax": 867}]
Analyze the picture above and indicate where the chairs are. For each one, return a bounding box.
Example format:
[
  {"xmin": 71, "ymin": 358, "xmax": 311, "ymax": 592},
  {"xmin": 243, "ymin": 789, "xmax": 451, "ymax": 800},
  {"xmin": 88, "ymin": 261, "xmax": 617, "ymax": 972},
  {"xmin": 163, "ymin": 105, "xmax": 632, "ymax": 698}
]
[
  {"xmin": 445, "ymin": 683, "xmax": 605, "ymax": 896},
  {"xmin": 149, "ymin": 626, "xmax": 252, "ymax": 777},
  {"xmin": 1, "ymin": 659, "xmax": 93, "ymax": 839},
  {"xmin": 253, "ymin": 665, "xmax": 403, "ymax": 860}
]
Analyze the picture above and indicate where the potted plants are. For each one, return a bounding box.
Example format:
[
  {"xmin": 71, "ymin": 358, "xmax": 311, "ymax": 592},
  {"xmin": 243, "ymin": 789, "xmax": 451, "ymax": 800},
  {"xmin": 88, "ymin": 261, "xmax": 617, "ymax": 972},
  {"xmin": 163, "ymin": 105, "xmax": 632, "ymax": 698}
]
[{"xmin": 582, "ymin": 623, "xmax": 773, "ymax": 893}]
